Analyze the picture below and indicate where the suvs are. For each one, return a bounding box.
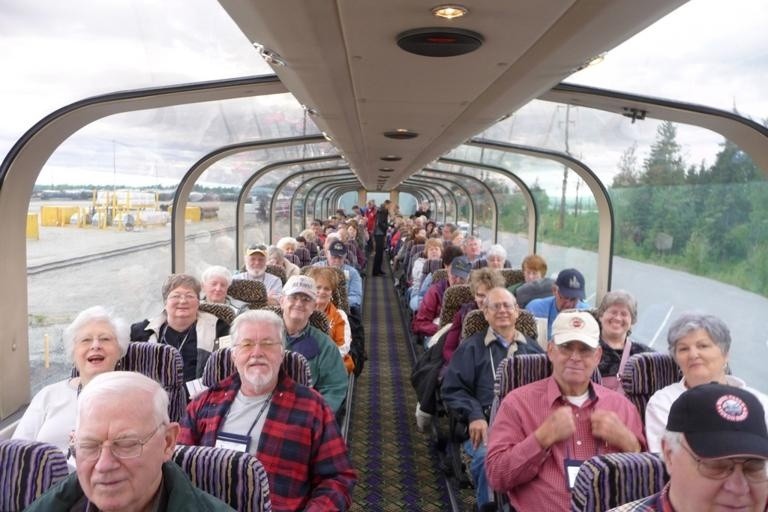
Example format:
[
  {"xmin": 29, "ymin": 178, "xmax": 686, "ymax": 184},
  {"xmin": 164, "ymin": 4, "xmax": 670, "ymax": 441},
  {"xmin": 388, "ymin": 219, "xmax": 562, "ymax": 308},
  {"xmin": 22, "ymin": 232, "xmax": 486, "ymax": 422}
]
[{"xmin": 264, "ymin": 197, "xmax": 315, "ymax": 220}]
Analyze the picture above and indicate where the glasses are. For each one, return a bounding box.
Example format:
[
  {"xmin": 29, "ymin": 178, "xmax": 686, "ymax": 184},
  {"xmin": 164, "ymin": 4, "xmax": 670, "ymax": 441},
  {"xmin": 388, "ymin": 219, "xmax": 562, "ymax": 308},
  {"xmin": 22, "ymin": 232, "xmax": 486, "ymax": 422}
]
[
  {"xmin": 556, "ymin": 345, "xmax": 597, "ymax": 356},
  {"xmin": 676, "ymin": 439, "xmax": 763, "ymax": 482},
  {"xmin": 233, "ymin": 340, "xmax": 284, "ymax": 352},
  {"xmin": 282, "ymin": 295, "xmax": 315, "ymax": 301},
  {"xmin": 168, "ymin": 293, "xmax": 199, "ymax": 301},
  {"xmin": 248, "ymin": 244, "xmax": 266, "ymax": 250},
  {"xmin": 75, "ymin": 421, "xmax": 172, "ymax": 461},
  {"xmin": 487, "ymin": 301, "xmax": 516, "ymax": 312}
]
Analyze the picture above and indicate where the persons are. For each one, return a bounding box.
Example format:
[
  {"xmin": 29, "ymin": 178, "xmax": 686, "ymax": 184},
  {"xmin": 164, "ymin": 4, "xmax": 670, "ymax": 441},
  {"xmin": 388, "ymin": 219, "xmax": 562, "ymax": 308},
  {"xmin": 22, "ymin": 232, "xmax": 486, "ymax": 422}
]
[
  {"xmin": 372, "ymin": 199, "xmax": 392, "ymax": 276},
  {"xmin": 10, "ymin": 268, "xmax": 359, "ymax": 512},
  {"xmin": 233, "ymin": 201, "xmax": 378, "ymax": 359},
  {"xmin": 441, "ymin": 267, "xmax": 768, "ymax": 511},
  {"xmin": 387, "ymin": 199, "xmax": 547, "ymax": 341}
]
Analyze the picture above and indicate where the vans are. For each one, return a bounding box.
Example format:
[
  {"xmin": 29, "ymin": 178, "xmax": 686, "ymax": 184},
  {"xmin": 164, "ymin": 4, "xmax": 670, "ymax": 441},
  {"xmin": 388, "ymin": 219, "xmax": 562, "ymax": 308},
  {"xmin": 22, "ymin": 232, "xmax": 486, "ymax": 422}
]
[{"xmin": 458, "ymin": 224, "xmax": 479, "ymax": 239}]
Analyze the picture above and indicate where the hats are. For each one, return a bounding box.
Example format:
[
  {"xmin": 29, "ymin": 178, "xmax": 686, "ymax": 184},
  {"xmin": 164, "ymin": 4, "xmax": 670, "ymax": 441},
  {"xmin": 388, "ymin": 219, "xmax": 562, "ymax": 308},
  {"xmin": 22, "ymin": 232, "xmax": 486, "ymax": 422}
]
[
  {"xmin": 244, "ymin": 241, "xmax": 270, "ymax": 257},
  {"xmin": 556, "ymin": 269, "xmax": 585, "ymax": 301},
  {"xmin": 665, "ymin": 378, "xmax": 764, "ymax": 459},
  {"xmin": 328, "ymin": 238, "xmax": 346, "ymax": 258},
  {"xmin": 449, "ymin": 255, "xmax": 472, "ymax": 279},
  {"xmin": 282, "ymin": 276, "xmax": 318, "ymax": 299},
  {"xmin": 552, "ymin": 308, "xmax": 602, "ymax": 348}
]
